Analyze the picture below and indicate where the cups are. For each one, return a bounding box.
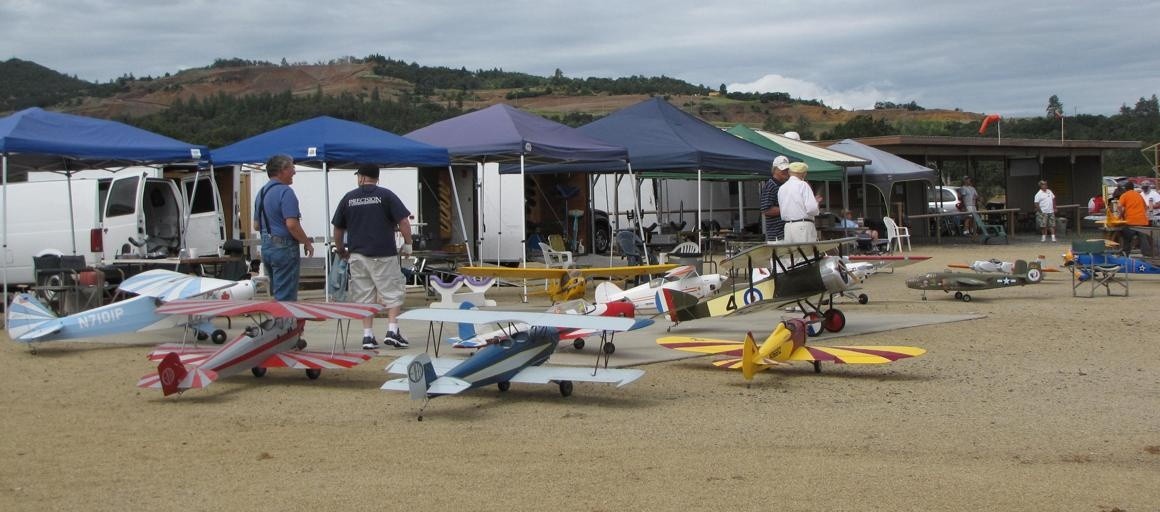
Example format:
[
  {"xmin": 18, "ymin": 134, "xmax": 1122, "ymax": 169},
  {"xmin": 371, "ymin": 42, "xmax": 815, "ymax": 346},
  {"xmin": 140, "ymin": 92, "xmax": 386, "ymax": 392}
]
[
  {"xmin": 659, "ymin": 252, "xmax": 667, "ymax": 265},
  {"xmin": 189, "ymin": 248, "xmax": 199, "ymax": 259},
  {"xmin": 179, "ymin": 249, "xmax": 187, "ymax": 258}
]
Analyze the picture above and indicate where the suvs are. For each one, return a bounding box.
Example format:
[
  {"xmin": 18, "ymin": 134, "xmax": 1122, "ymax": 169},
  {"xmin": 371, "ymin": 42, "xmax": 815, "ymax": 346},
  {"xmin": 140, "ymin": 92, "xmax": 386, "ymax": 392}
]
[{"xmin": 926, "ymin": 180, "xmax": 981, "ymax": 225}]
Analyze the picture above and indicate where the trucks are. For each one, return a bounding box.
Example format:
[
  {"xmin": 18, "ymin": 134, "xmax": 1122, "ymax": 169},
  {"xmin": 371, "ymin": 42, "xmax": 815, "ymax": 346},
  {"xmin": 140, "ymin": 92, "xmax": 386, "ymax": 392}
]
[{"xmin": 0, "ymin": 172, "xmax": 230, "ymax": 305}]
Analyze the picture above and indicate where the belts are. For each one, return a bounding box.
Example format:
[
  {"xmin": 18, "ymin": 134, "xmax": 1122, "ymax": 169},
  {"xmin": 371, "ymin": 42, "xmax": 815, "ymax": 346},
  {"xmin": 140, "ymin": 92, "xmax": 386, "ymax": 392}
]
[{"xmin": 783, "ymin": 218, "xmax": 815, "ymax": 224}]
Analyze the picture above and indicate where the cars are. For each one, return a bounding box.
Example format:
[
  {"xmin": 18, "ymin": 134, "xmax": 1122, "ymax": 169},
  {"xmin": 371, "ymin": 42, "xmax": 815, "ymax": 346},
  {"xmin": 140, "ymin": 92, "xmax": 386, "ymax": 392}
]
[
  {"xmin": 590, "ymin": 203, "xmax": 617, "ymax": 255},
  {"xmin": 1103, "ymin": 174, "xmax": 1160, "ymax": 207}
]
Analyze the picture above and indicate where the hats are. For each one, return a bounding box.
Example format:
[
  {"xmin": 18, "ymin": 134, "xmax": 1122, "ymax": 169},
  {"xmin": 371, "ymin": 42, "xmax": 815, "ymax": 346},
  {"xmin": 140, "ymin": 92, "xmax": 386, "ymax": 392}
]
[
  {"xmin": 773, "ymin": 155, "xmax": 790, "ymax": 170},
  {"xmin": 354, "ymin": 162, "xmax": 380, "ymax": 179},
  {"xmin": 788, "ymin": 161, "xmax": 808, "ymax": 175},
  {"xmin": 840, "ymin": 208, "xmax": 852, "ymax": 216},
  {"xmin": 1140, "ymin": 180, "xmax": 1151, "ymax": 188}
]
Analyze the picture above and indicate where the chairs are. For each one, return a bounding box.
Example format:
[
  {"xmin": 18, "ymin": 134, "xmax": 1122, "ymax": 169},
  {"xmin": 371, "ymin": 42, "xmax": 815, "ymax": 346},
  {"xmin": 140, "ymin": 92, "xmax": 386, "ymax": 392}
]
[
  {"xmin": 883, "ymin": 217, "xmax": 911, "ymax": 253},
  {"xmin": 538, "ymin": 241, "xmax": 577, "ymax": 292},
  {"xmin": 1071, "ymin": 238, "xmax": 1129, "ymax": 298},
  {"xmin": 1014, "ymin": 207, "xmax": 1036, "ymax": 233},
  {"xmin": 617, "ymin": 230, "xmax": 659, "ymax": 290},
  {"xmin": 32, "ymin": 254, "xmax": 125, "ymax": 316},
  {"xmin": 659, "ymin": 242, "xmax": 699, "ymax": 265},
  {"xmin": 702, "ymin": 237, "xmax": 717, "ymax": 274},
  {"xmin": 193, "ymin": 239, "xmax": 271, "ymax": 298},
  {"xmin": 548, "ymin": 232, "xmax": 597, "ymax": 290},
  {"xmin": 859, "ymin": 233, "xmax": 888, "ymax": 252}
]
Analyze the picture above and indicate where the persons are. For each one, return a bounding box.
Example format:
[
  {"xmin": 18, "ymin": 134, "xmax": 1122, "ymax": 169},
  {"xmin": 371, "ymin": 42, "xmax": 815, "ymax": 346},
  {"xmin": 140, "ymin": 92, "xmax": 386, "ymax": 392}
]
[
  {"xmin": 1034, "ymin": 180, "xmax": 1057, "ymax": 242},
  {"xmin": 1121, "ymin": 181, "xmax": 1152, "ymax": 257},
  {"xmin": 960, "ymin": 176, "xmax": 980, "ymax": 236},
  {"xmin": 1140, "ymin": 180, "xmax": 1160, "ymax": 216},
  {"xmin": 841, "ymin": 209, "xmax": 879, "ymax": 251},
  {"xmin": 254, "ymin": 155, "xmax": 314, "ymax": 350},
  {"xmin": 760, "ymin": 156, "xmax": 789, "ymax": 242},
  {"xmin": 778, "ymin": 162, "xmax": 823, "ymax": 241},
  {"xmin": 331, "ymin": 164, "xmax": 413, "ymax": 349}
]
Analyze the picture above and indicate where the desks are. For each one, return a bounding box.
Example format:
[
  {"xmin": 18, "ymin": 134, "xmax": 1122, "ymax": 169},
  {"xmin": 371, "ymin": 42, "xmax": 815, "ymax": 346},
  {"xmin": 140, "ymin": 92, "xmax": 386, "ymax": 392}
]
[
  {"xmin": 100, "ymin": 256, "xmax": 240, "ymax": 277},
  {"xmin": 816, "ymin": 226, "xmax": 870, "ymax": 256},
  {"xmin": 397, "ymin": 247, "xmax": 465, "ymax": 284}
]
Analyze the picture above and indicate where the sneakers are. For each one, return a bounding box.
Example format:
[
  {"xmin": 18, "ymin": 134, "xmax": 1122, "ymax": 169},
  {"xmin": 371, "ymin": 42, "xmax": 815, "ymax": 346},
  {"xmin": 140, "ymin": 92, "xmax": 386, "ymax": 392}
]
[
  {"xmin": 383, "ymin": 326, "xmax": 409, "ymax": 347},
  {"xmin": 1040, "ymin": 237, "xmax": 1057, "ymax": 242},
  {"xmin": 361, "ymin": 335, "xmax": 380, "ymax": 349}
]
